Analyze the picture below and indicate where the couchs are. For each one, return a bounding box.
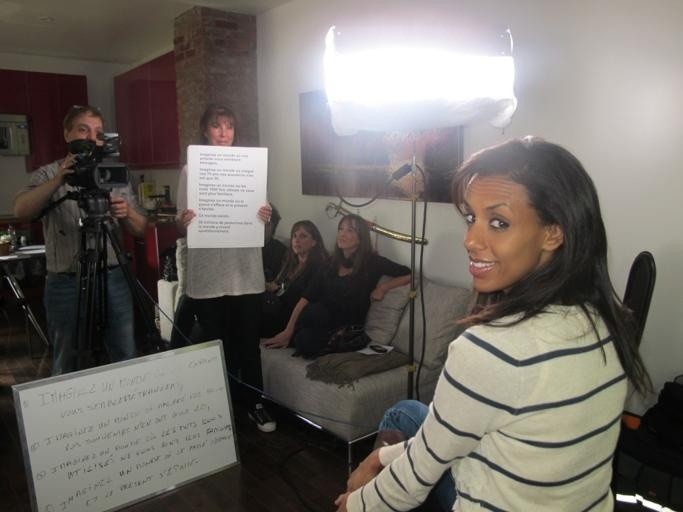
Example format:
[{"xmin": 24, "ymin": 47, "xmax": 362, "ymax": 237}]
[{"xmin": 174, "ymin": 236, "xmax": 471, "ymax": 480}]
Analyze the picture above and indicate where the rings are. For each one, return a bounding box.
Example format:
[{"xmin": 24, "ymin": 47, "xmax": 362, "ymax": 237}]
[{"xmin": 61, "ymin": 163, "xmax": 66, "ymax": 169}]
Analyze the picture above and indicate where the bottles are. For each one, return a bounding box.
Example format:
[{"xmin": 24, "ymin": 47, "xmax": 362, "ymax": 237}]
[
  {"xmin": 7, "ymin": 224, "xmax": 17, "ymax": 246},
  {"xmin": 20, "ymin": 233, "xmax": 27, "ymax": 247}
]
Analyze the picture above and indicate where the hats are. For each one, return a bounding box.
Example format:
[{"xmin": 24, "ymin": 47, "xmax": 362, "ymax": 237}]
[{"xmin": 55, "ymin": 104, "xmax": 102, "ymax": 149}]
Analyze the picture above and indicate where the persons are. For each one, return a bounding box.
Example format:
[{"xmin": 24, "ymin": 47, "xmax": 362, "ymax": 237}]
[
  {"xmin": 260, "ymin": 212, "xmax": 413, "ymax": 362},
  {"xmin": 167, "ymin": 100, "xmax": 281, "ymax": 435},
  {"xmin": 12, "ymin": 102, "xmax": 152, "ymax": 380},
  {"xmin": 259, "ymin": 217, "xmax": 329, "ymax": 339},
  {"xmin": 261, "ymin": 199, "xmax": 288, "ymax": 284},
  {"xmin": 329, "ymin": 131, "xmax": 652, "ymax": 512}
]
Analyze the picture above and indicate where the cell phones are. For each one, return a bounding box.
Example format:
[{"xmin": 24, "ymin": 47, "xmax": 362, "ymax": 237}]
[{"xmin": 370, "ymin": 345, "xmax": 387, "ymax": 353}]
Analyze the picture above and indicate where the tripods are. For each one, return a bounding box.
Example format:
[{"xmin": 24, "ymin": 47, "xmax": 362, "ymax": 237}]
[{"xmin": 72, "ymin": 216, "xmax": 168, "ymax": 372}]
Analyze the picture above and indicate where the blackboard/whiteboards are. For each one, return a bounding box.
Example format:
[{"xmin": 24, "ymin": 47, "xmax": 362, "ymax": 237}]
[{"xmin": 11, "ymin": 338, "xmax": 241, "ymax": 512}]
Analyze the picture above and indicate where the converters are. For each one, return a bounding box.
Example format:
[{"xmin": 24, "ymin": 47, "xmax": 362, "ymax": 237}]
[{"xmin": 392, "ymin": 164, "xmax": 412, "ymax": 181}]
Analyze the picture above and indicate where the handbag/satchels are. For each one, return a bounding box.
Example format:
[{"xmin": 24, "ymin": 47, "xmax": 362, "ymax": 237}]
[
  {"xmin": 330, "ymin": 324, "xmax": 366, "ymax": 349},
  {"xmin": 303, "ymin": 337, "xmax": 333, "ymax": 358}
]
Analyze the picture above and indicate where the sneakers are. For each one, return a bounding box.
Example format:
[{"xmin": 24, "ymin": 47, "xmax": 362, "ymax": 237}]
[{"xmin": 246, "ymin": 405, "xmax": 278, "ymax": 433}]
